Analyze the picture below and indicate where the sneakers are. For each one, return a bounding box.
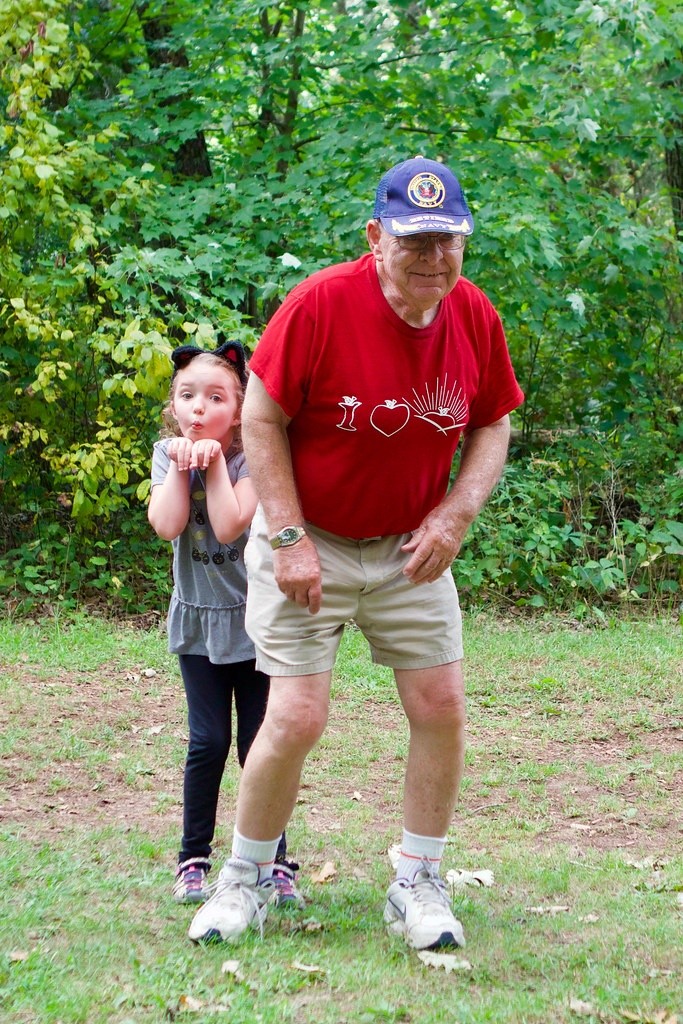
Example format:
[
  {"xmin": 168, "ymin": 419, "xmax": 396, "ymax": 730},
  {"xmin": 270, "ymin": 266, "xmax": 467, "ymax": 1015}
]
[
  {"xmin": 384, "ymin": 855, "xmax": 468, "ymax": 953},
  {"xmin": 189, "ymin": 859, "xmax": 266, "ymax": 948},
  {"xmin": 272, "ymin": 864, "xmax": 305, "ymax": 910},
  {"xmin": 174, "ymin": 856, "xmax": 210, "ymax": 903}
]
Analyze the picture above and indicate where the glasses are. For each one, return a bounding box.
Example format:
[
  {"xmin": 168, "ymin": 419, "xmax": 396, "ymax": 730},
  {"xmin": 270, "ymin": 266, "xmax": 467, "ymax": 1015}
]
[{"xmin": 396, "ymin": 234, "xmax": 465, "ymax": 251}]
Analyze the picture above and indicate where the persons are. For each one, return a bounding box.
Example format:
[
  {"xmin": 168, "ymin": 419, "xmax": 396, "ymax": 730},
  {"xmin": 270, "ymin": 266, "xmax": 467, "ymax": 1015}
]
[
  {"xmin": 147, "ymin": 337, "xmax": 308, "ymax": 913},
  {"xmin": 188, "ymin": 156, "xmax": 527, "ymax": 951}
]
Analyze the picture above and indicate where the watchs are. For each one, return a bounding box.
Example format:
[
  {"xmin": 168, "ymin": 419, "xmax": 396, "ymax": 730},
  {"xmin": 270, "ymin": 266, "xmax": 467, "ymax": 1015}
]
[{"xmin": 269, "ymin": 524, "xmax": 308, "ymax": 552}]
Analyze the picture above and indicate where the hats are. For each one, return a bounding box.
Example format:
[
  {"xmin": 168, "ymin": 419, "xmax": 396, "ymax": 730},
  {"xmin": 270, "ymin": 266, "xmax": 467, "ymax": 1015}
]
[{"xmin": 374, "ymin": 156, "xmax": 476, "ymax": 238}]
